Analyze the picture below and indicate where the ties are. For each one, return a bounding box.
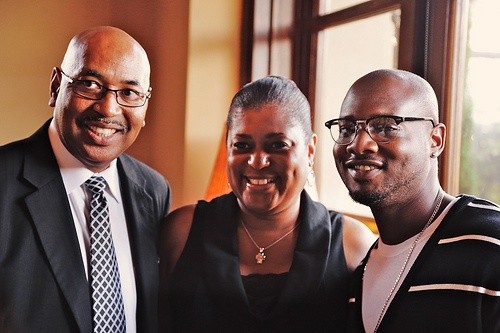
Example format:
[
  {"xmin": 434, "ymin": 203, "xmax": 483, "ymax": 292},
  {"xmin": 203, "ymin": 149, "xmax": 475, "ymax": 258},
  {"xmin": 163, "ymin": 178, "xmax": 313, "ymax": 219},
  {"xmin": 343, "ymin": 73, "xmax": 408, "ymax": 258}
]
[{"xmin": 84, "ymin": 177, "xmax": 127, "ymax": 333}]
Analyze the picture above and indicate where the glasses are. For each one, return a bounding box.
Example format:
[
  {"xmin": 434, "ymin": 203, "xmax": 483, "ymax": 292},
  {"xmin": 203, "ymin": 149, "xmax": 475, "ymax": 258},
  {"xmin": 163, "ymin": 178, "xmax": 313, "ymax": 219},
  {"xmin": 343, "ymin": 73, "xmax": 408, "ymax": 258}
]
[
  {"xmin": 325, "ymin": 116, "xmax": 437, "ymax": 146},
  {"xmin": 59, "ymin": 70, "xmax": 150, "ymax": 107}
]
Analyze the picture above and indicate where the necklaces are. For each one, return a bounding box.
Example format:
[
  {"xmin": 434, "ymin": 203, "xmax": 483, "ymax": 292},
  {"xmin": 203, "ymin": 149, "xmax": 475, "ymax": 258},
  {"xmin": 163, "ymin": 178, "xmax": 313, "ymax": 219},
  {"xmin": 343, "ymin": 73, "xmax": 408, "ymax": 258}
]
[
  {"xmin": 362, "ymin": 192, "xmax": 444, "ymax": 332},
  {"xmin": 240, "ymin": 214, "xmax": 299, "ymax": 264}
]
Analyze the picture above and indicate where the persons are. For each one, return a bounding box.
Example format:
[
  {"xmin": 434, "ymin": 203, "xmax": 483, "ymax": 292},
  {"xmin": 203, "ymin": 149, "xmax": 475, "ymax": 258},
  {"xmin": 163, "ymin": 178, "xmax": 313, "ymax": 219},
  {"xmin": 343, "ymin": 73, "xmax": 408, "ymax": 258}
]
[
  {"xmin": 0, "ymin": 26, "xmax": 173, "ymax": 333},
  {"xmin": 324, "ymin": 68, "xmax": 500, "ymax": 333},
  {"xmin": 163, "ymin": 77, "xmax": 374, "ymax": 333}
]
